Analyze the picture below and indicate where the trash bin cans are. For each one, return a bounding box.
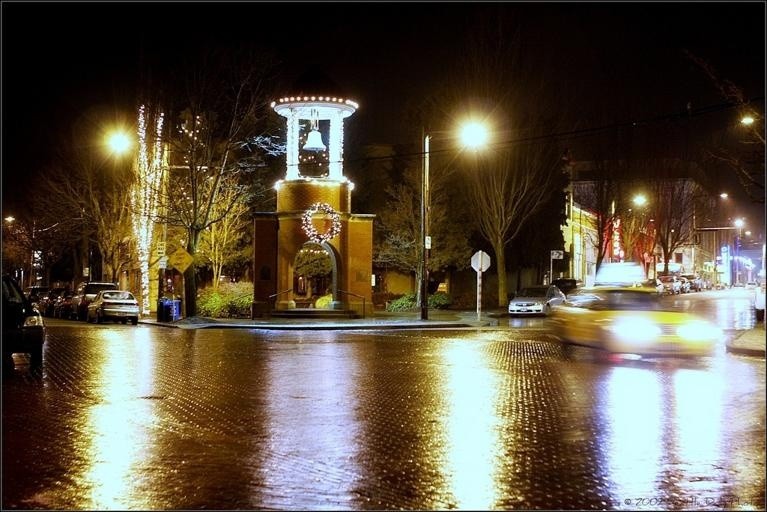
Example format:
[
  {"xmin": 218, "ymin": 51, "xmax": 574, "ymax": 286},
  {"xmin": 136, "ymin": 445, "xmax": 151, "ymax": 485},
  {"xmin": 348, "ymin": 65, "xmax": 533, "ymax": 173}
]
[{"xmin": 156, "ymin": 298, "xmax": 182, "ymax": 322}]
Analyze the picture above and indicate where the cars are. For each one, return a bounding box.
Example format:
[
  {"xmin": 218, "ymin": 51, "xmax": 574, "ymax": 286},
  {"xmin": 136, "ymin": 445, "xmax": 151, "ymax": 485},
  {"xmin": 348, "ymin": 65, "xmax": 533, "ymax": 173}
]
[
  {"xmin": 552, "ymin": 274, "xmax": 705, "ymax": 294},
  {"xmin": 0, "ymin": 276, "xmax": 44, "ymax": 368},
  {"xmin": 552, "ymin": 288, "xmax": 725, "ymax": 359},
  {"xmin": 744, "ymin": 282, "xmax": 766, "ymax": 321},
  {"xmin": 23, "ymin": 283, "xmax": 137, "ymax": 325},
  {"xmin": 507, "ymin": 285, "xmax": 568, "ymax": 317}
]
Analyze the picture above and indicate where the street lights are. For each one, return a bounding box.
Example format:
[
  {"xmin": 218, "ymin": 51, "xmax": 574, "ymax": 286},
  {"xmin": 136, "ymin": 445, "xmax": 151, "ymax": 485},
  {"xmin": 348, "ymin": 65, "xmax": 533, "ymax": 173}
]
[
  {"xmin": 731, "ymin": 231, "xmax": 751, "ymax": 287},
  {"xmin": 109, "ymin": 133, "xmax": 196, "ymax": 317},
  {"xmin": 611, "ymin": 195, "xmax": 645, "ymax": 263},
  {"xmin": 421, "ymin": 126, "xmax": 488, "ymax": 321},
  {"xmin": 690, "ymin": 191, "xmax": 729, "ymax": 274}
]
